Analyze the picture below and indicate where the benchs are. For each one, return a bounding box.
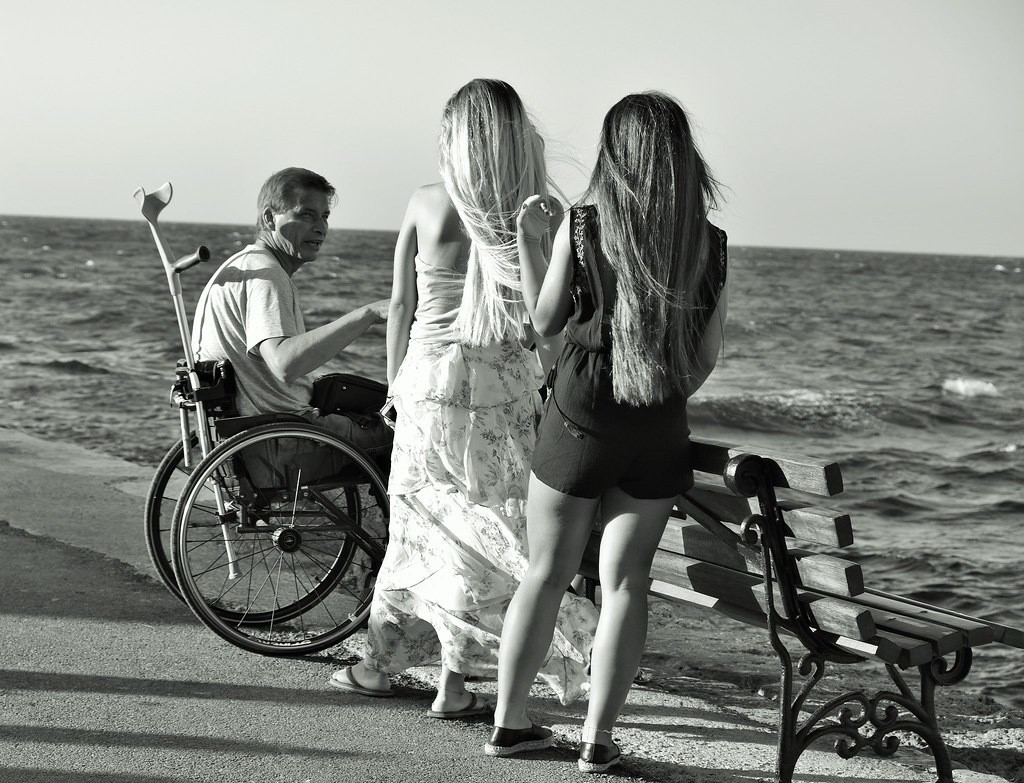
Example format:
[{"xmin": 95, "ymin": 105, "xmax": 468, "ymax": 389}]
[{"xmin": 567, "ymin": 417, "xmax": 1024, "ymax": 782}]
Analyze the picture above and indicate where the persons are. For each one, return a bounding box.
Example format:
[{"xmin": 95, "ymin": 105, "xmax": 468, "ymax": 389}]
[
  {"xmin": 486, "ymin": 90, "xmax": 729, "ymax": 775},
  {"xmin": 189, "ymin": 166, "xmax": 406, "ymax": 537},
  {"xmin": 329, "ymin": 78, "xmax": 600, "ymax": 716}
]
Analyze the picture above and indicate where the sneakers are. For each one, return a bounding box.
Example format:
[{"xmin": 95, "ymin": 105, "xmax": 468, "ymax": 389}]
[
  {"xmin": 485, "ymin": 724, "xmax": 553, "ymax": 757},
  {"xmin": 577, "ymin": 741, "xmax": 622, "ymax": 772}
]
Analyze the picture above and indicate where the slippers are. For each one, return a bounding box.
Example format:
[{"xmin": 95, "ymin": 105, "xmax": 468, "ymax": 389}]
[
  {"xmin": 426, "ymin": 689, "xmax": 488, "ymax": 719},
  {"xmin": 328, "ymin": 666, "xmax": 395, "ymax": 696}
]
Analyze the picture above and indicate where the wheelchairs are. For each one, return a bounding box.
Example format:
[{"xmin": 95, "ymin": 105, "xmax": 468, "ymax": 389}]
[{"xmin": 127, "ymin": 181, "xmax": 397, "ymax": 658}]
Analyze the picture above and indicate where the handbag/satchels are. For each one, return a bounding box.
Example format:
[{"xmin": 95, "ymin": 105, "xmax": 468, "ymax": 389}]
[{"xmin": 311, "ymin": 372, "xmax": 397, "ymax": 430}]
